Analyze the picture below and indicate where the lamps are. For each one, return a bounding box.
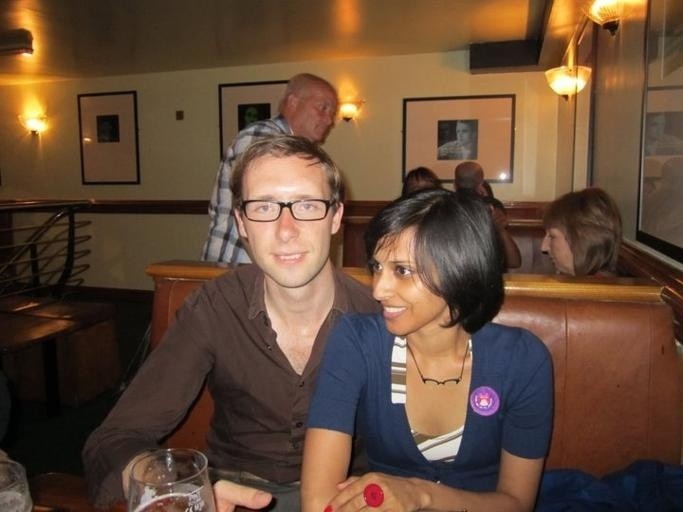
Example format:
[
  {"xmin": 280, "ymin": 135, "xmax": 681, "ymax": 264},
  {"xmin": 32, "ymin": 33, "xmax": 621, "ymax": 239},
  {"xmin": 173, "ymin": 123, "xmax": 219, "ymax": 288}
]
[
  {"xmin": 17, "ymin": 113, "xmax": 49, "ymax": 136},
  {"xmin": 580, "ymin": 0, "xmax": 624, "ymax": 37},
  {"xmin": 543, "ymin": 65, "xmax": 593, "ymax": 102},
  {"xmin": 337, "ymin": 101, "xmax": 361, "ymax": 122}
]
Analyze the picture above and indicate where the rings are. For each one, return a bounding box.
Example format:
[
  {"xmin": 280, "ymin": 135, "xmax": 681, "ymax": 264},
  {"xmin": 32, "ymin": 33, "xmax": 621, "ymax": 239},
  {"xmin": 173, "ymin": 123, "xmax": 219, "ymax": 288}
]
[{"xmin": 364, "ymin": 483, "xmax": 384, "ymax": 508}]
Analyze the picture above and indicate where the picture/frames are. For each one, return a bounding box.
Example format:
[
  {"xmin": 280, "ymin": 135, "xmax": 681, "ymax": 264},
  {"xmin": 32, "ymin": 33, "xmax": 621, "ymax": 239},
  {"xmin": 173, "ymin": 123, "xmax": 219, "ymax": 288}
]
[
  {"xmin": 635, "ymin": 0, "xmax": 682, "ymax": 265},
  {"xmin": 402, "ymin": 93, "xmax": 516, "ymax": 183},
  {"xmin": 643, "ymin": 86, "xmax": 682, "ymax": 179},
  {"xmin": 218, "ymin": 80, "xmax": 291, "ymax": 161},
  {"xmin": 77, "ymin": 90, "xmax": 141, "ymax": 185}
]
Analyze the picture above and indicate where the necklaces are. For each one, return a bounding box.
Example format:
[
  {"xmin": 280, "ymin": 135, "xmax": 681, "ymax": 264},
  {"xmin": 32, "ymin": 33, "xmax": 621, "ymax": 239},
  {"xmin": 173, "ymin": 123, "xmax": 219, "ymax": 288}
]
[{"xmin": 407, "ymin": 343, "xmax": 469, "ymax": 386}]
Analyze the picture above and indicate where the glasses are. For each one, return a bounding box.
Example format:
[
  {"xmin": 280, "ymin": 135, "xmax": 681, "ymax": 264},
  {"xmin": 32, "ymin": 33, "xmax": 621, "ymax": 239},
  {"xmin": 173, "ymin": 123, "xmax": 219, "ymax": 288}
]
[{"xmin": 238, "ymin": 197, "xmax": 337, "ymax": 223}]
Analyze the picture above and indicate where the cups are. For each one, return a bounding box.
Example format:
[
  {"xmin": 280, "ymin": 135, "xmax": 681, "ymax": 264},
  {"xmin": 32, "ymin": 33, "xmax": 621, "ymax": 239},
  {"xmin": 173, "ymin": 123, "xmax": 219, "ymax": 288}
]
[
  {"xmin": 128, "ymin": 449, "xmax": 215, "ymax": 511},
  {"xmin": 0, "ymin": 458, "xmax": 32, "ymax": 511}
]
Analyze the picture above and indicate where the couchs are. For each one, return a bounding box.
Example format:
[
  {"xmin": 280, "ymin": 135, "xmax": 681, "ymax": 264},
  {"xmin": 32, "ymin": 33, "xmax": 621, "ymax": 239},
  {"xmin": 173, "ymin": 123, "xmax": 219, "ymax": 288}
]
[
  {"xmin": 339, "ymin": 218, "xmax": 558, "ymax": 275},
  {"xmin": 146, "ymin": 260, "xmax": 682, "ymax": 474}
]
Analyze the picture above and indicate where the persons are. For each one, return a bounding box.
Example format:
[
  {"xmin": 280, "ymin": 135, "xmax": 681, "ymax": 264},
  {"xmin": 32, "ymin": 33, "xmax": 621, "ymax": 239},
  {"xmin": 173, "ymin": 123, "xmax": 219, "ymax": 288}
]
[
  {"xmin": 403, "ymin": 167, "xmax": 522, "ymax": 268},
  {"xmin": 454, "ymin": 163, "xmax": 507, "ymax": 214},
  {"xmin": 82, "ymin": 136, "xmax": 383, "ymax": 512},
  {"xmin": 101, "ymin": 121, "xmax": 114, "ymax": 138},
  {"xmin": 302, "ymin": 189, "xmax": 554, "ymax": 512},
  {"xmin": 540, "ymin": 190, "xmax": 623, "ymax": 279},
  {"xmin": 202, "ymin": 73, "xmax": 337, "ymax": 264},
  {"xmin": 438, "ymin": 120, "xmax": 477, "ymax": 160},
  {"xmin": 244, "ymin": 105, "xmax": 259, "ymax": 126},
  {"xmin": 480, "ymin": 182, "xmax": 492, "ymax": 197},
  {"xmin": 641, "ymin": 158, "xmax": 682, "ymax": 246}
]
